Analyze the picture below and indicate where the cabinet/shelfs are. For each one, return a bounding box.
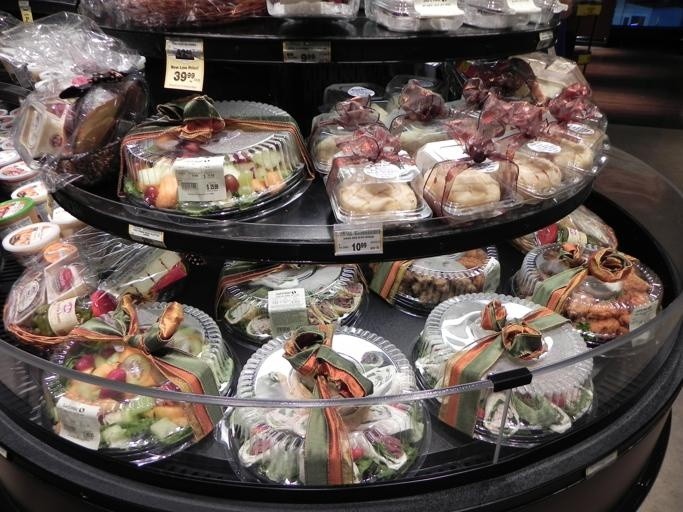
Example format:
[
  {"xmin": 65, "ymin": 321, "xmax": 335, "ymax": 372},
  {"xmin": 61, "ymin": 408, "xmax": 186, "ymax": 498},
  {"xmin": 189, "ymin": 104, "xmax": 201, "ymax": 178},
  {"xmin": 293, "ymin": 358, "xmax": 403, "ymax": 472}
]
[{"xmin": 0, "ymin": 14, "xmax": 683, "ymax": 512}]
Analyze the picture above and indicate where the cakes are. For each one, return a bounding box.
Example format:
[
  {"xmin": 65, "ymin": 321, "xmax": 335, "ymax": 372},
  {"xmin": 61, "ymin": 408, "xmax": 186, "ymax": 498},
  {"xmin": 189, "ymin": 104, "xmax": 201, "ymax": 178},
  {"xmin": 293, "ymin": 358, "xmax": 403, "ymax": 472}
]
[
  {"xmin": 221, "ymin": 259, "xmax": 363, "ymax": 337},
  {"xmin": 426, "ymin": 164, "xmax": 500, "ymax": 208},
  {"xmin": 369, "ymin": 247, "xmax": 494, "ymax": 303},
  {"xmin": 388, "ymin": 109, "xmax": 447, "ymax": 159},
  {"xmin": 333, "ymin": 160, "xmax": 417, "ymax": 220},
  {"xmin": 123, "ymin": 101, "xmax": 301, "ymax": 212},
  {"xmin": 414, "ymin": 304, "xmax": 593, "ymax": 439},
  {"xmin": 547, "ymin": 122, "xmax": 605, "ymax": 172},
  {"xmin": 234, "ymin": 344, "xmax": 424, "ymax": 484},
  {"xmin": 52, "ymin": 321, "xmax": 236, "ymax": 447},
  {"xmin": 518, "ymin": 247, "xmax": 652, "ymax": 336},
  {"xmin": 496, "ymin": 152, "xmax": 560, "ymax": 200},
  {"xmin": 314, "ymin": 111, "xmax": 386, "ymax": 166}
]
